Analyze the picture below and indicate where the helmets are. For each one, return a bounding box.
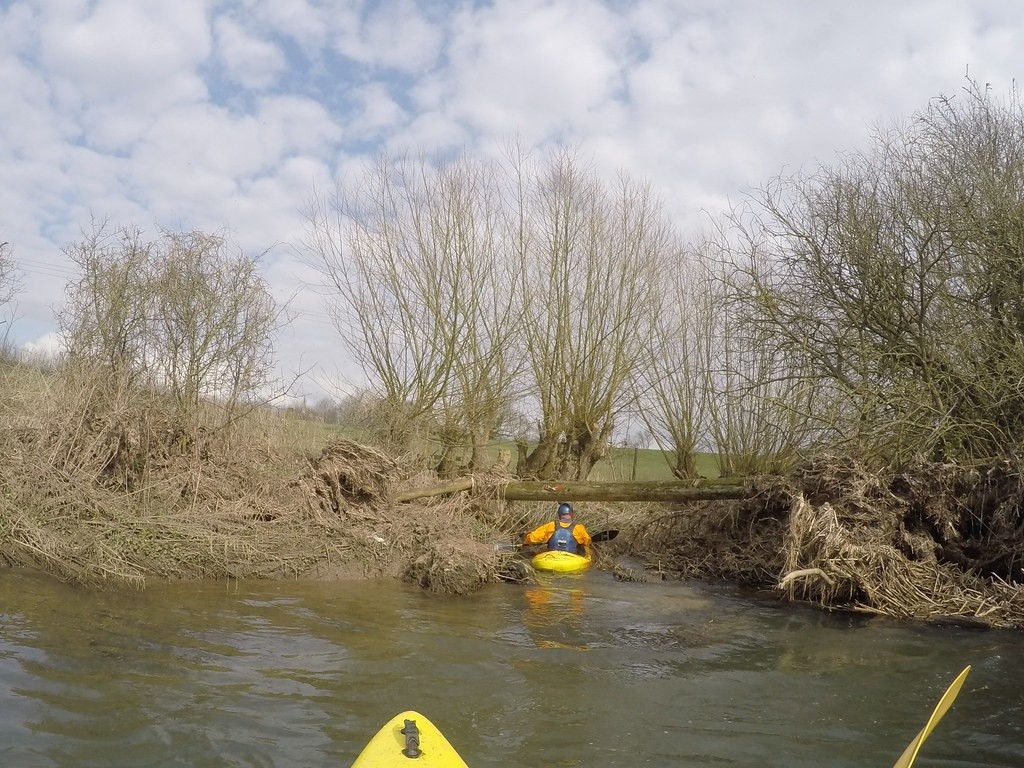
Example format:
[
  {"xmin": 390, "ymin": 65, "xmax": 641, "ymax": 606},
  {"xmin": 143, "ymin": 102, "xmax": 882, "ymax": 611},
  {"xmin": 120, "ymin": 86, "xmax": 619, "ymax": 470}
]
[{"xmin": 558, "ymin": 503, "xmax": 573, "ymax": 516}]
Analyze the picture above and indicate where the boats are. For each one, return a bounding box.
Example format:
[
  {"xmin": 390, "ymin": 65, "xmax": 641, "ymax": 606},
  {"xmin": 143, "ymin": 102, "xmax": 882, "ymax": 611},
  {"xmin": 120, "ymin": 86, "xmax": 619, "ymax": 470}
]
[{"xmin": 530, "ymin": 541, "xmax": 594, "ymax": 573}]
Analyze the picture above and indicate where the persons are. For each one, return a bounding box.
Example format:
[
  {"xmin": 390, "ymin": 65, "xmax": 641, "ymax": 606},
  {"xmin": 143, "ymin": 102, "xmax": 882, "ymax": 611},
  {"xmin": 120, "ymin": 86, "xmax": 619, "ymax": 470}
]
[{"xmin": 526, "ymin": 503, "xmax": 591, "ymax": 555}]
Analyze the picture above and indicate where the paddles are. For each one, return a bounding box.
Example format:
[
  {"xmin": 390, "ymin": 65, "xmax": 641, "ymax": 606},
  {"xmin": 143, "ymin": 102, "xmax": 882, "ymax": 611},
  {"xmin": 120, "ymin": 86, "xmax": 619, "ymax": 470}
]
[
  {"xmin": 891, "ymin": 663, "xmax": 971, "ymax": 768},
  {"xmin": 496, "ymin": 530, "xmax": 619, "ymax": 548}
]
